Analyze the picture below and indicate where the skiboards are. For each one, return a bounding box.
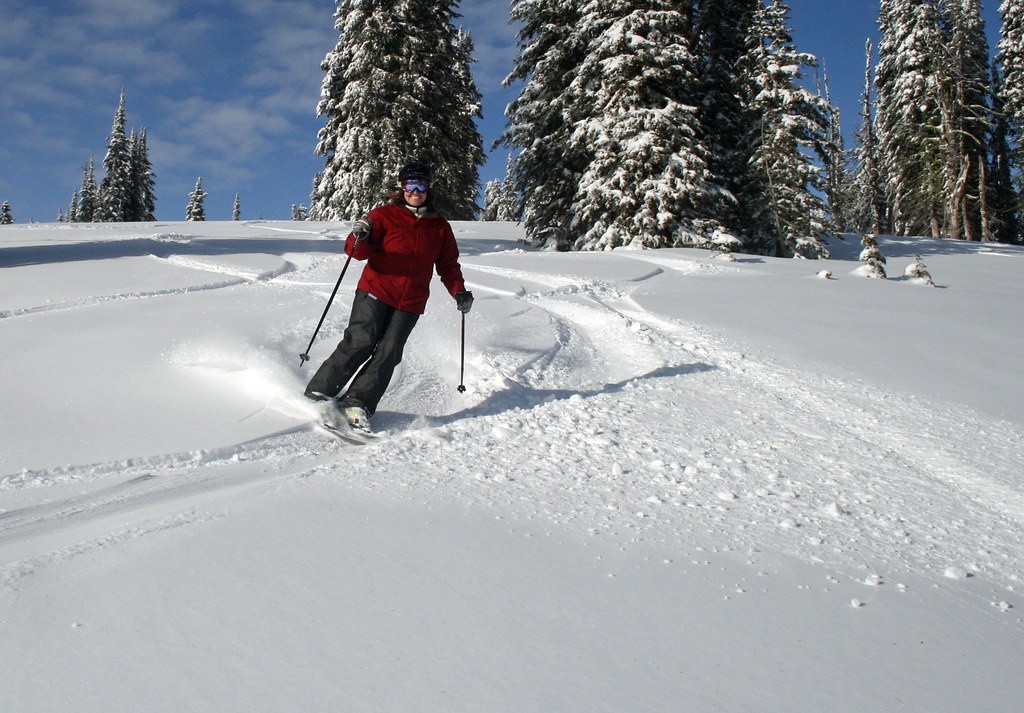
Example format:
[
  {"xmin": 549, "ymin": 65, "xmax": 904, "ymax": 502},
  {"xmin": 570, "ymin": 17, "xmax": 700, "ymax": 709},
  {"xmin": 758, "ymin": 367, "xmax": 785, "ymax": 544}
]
[{"xmin": 306, "ymin": 401, "xmax": 386, "ymax": 444}]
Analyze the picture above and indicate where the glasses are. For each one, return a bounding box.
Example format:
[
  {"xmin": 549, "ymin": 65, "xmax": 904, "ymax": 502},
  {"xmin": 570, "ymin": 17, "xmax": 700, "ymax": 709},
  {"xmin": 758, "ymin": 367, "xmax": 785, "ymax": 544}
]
[{"xmin": 397, "ymin": 178, "xmax": 434, "ymax": 193}]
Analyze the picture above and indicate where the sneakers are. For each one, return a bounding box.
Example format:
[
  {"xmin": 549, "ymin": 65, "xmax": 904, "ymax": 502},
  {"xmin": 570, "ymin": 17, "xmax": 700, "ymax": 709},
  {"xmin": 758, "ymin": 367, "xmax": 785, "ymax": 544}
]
[
  {"xmin": 304, "ymin": 391, "xmax": 340, "ymax": 429},
  {"xmin": 337, "ymin": 398, "xmax": 372, "ymax": 431}
]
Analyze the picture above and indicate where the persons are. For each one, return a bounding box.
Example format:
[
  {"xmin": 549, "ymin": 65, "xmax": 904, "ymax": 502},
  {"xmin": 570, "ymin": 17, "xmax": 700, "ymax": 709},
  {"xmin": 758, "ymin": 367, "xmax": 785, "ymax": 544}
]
[{"xmin": 303, "ymin": 161, "xmax": 473, "ymax": 435}]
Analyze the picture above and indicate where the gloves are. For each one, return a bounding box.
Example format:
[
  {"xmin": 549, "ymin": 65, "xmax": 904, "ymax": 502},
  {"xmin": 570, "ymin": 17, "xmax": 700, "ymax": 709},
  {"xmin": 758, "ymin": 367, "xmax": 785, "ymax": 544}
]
[
  {"xmin": 455, "ymin": 291, "xmax": 474, "ymax": 313},
  {"xmin": 352, "ymin": 217, "xmax": 372, "ymax": 240}
]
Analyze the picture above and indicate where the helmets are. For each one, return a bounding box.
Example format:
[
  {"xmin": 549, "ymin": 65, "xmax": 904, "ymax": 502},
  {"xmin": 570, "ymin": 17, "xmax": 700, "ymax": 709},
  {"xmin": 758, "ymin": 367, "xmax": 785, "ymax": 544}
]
[{"xmin": 399, "ymin": 162, "xmax": 433, "ymax": 182}]
